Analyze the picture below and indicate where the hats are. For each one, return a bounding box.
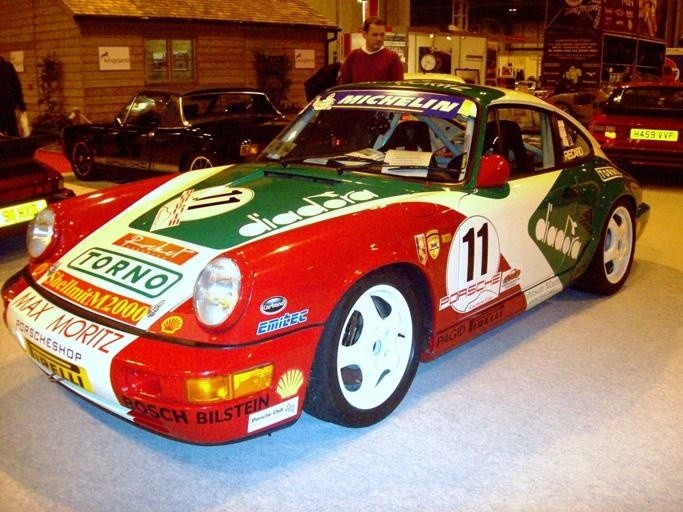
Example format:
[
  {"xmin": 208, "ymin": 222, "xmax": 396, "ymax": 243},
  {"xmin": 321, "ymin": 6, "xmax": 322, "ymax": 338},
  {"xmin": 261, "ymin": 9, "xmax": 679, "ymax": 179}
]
[
  {"xmin": 547, "ymin": 91, "xmax": 609, "ymax": 116},
  {"xmin": 588, "ymin": 82, "xmax": 683, "ymax": 169},
  {"xmin": 1, "ymin": 79, "xmax": 642, "ymax": 444},
  {"xmin": 62, "ymin": 88, "xmax": 291, "ymax": 181}
]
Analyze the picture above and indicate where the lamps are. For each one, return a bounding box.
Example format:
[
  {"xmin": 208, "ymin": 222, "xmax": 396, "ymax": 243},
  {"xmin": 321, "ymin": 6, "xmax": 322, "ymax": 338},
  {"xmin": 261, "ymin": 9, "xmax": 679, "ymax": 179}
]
[
  {"xmin": 181, "ymin": 103, "xmax": 200, "ymax": 117},
  {"xmin": 226, "ymin": 102, "xmax": 248, "ymax": 113},
  {"xmin": 374, "ymin": 121, "xmax": 440, "ymax": 170},
  {"xmin": 639, "ymin": 93, "xmax": 683, "ymax": 109},
  {"xmin": 482, "ymin": 119, "xmax": 535, "ymax": 172},
  {"xmin": 136, "ymin": 111, "xmax": 160, "ymax": 127}
]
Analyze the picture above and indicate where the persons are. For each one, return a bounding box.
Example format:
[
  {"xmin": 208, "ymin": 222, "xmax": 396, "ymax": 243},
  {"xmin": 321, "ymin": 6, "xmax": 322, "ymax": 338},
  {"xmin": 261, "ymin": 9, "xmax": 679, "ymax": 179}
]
[
  {"xmin": 333, "ymin": 15, "xmax": 403, "ymax": 147},
  {"xmin": 0, "ymin": 56, "xmax": 33, "ymax": 139}
]
